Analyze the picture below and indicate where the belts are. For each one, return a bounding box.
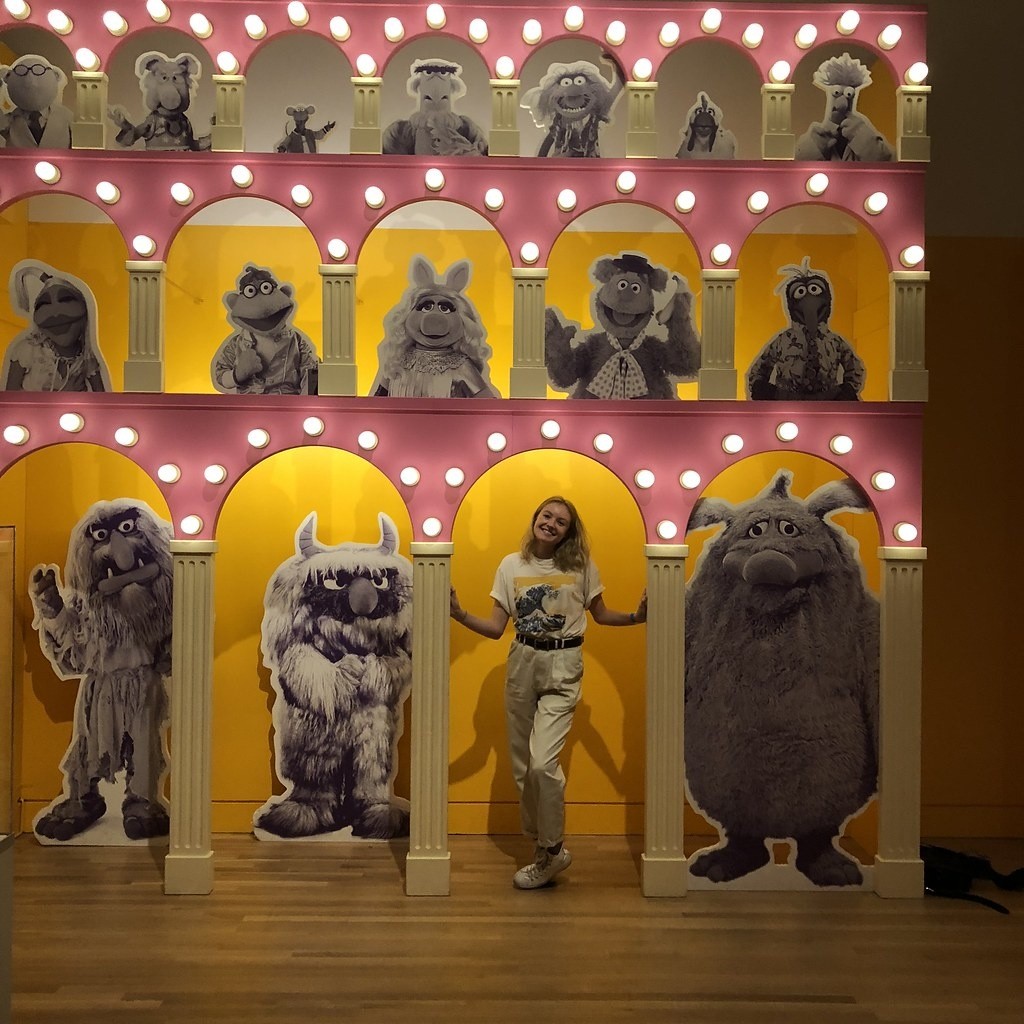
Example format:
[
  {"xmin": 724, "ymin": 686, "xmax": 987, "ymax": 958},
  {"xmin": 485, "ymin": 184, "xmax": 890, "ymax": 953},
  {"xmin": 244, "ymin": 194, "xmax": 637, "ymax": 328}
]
[{"xmin": 516, "ymin": 633, "xmax": 581, "ymax": 651}]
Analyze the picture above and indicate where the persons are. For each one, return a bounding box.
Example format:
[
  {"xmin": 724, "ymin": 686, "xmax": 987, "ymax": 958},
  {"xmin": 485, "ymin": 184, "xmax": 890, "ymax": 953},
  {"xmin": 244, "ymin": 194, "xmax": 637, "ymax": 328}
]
[{"xmin": 450, "ymin": 494, "xmax": 646, "ymax": 890}]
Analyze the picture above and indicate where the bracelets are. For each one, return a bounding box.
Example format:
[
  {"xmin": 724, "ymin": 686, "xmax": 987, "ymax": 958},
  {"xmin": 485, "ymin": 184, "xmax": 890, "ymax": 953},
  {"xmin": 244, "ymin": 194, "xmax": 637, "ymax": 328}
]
[
  {"xmin": 629, "ymin": 613, "xmax": 636, "ymax": 623},
  {"xmin": 460, "ymin": 611, "xmax": 468, "ymax": 624}
]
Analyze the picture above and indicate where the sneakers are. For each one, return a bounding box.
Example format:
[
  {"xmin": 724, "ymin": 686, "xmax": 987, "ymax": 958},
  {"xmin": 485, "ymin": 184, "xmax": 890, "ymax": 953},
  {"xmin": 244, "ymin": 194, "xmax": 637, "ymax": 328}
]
[{"xmin": 513, "ymin": 849, "xmax": 572, "ymax": 890}]
